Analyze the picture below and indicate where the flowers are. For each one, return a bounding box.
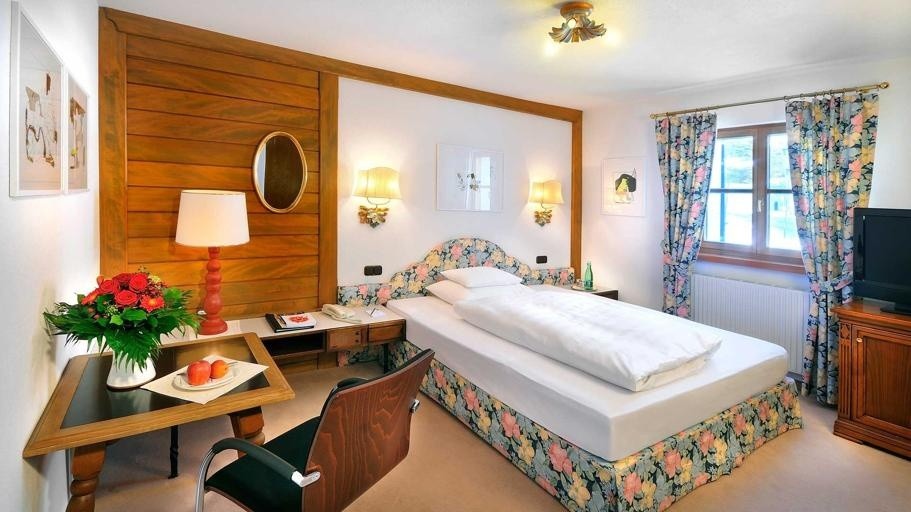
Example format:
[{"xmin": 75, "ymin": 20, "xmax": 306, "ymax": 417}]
[{"xmin": 43, "ymin": 269, "xmax": 212, "ymax": 374}]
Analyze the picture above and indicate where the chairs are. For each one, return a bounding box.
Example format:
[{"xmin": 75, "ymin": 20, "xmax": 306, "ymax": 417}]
[{"xmin": 190, "ymin": 346, "xmax": 436, "ymax": 512}]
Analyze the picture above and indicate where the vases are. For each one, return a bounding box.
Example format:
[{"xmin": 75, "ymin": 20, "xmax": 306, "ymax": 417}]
[{"xmin": 102, "ymin": 344, "xmax": 157, "ymax": 390}]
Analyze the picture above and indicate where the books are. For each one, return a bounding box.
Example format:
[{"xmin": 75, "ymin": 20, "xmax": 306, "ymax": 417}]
[{"xmin": 264, "ymin": 310, "xmax": 316, "ymax": 332}]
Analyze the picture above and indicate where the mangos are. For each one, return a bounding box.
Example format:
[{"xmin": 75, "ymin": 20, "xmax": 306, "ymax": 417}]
[{"xmin": 211, "ymin": 359, "xmax": 228, "ymax": 378}]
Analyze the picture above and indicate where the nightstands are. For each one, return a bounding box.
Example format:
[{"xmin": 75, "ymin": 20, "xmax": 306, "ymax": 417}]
[{"xmin": 564, "ymin": 276, "xmax": 622, "ymax": 300}]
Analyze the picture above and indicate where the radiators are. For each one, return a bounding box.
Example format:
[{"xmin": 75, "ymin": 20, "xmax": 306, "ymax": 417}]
[{"xmin": 686, "ymin": 272, "xmax": 813, "ymax": 379}]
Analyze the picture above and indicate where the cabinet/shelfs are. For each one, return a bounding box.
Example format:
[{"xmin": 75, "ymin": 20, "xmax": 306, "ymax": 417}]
[
  {"xmin": 260, "ymin": 305, "xmax": 408, "ymax": 375},
  {"xmin": 827, "ymin": 297, "xmax": 911, "ymax": 464}
]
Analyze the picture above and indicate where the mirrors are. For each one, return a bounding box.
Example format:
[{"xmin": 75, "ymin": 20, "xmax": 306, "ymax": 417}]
[{"xmin": 250, "ymin": 129, "xmax": 310, "ymax": 214}]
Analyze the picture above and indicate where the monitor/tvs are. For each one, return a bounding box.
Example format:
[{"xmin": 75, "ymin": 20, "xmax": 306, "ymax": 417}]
[{"xmin": 853, "ymin": 207, "xmax": 911, "ymax": 315}]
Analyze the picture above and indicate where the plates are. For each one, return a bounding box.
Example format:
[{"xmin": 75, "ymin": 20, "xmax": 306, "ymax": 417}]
[{"xmin": 173, "ymin": 367, "xmax": 241, "ymax": 390}]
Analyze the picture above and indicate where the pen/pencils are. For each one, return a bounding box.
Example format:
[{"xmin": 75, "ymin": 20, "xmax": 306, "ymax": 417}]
[{"xmin": 370, "ymin": 308, "xmax": 376, "ymax": 316}]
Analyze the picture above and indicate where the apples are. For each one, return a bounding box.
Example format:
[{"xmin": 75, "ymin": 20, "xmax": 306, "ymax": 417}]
[{"xmin": 188, "ymin": 360, "xmax": 212, "ymax": 386}]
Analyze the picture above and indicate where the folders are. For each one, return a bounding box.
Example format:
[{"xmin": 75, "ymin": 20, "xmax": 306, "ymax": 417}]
[{"xmin": 265, "ymin": 313, "xmax": 314, "ymax": 333}]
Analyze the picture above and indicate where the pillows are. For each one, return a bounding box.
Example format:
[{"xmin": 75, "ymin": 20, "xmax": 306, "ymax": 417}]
[{"xmin": 426, "ymin": 264, "xmax": 529, "ymax": 310}]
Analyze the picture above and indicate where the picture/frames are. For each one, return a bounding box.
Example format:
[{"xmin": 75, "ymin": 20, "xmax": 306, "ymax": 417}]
[
  {"xmin": 6, "ymin": 2, "xmax": 90, "ymax": 198},
  {"xmin": 599, "ymin": 155, "xmax": 648, "ymax": 218},
  {"xmin": 436, "ymin": 140, "xmax": 509, "ymax": 213}
]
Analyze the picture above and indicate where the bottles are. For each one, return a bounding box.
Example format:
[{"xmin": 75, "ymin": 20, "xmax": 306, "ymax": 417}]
[{"xmin": 584, "ymin": 261, "xmax": 594, "ymax": 290}]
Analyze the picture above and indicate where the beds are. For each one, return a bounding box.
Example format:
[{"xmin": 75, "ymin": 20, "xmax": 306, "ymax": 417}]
[{"xmin": 378, "ymin": 234, "xmax": 801, "ymax": 512}]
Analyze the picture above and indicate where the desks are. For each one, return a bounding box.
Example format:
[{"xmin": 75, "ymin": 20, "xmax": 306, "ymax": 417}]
[{"xmin": 20, "ymin": 329, "xmax": 296, "ymax": 512}]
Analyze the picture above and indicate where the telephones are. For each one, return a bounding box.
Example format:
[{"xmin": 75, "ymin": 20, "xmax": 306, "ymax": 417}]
[{"xmin": 323, "ymin": 303, "xmax": 354, "ymax": 320}]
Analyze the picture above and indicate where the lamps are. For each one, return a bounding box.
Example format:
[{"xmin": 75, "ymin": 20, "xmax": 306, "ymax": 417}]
[
  {"xmin": 173, "ymin": 188, "xmax": 254, "ymax": 338},
  {"xmin": 530, "ymin": 179, "xmax": 566, "ymax": 228},
  {"xmin": 548, "ymin": 0, "xmax": 608, "ymax": 50},
  {"xmin": 354, "ymin": 164, "xmax": 402, "ymax": 228}
]
